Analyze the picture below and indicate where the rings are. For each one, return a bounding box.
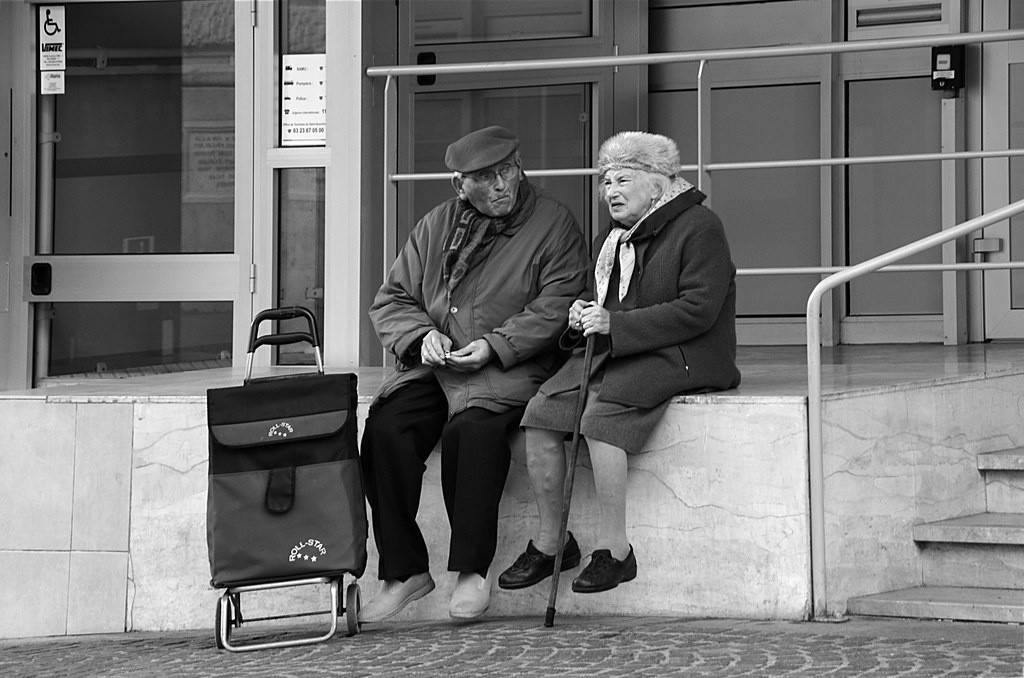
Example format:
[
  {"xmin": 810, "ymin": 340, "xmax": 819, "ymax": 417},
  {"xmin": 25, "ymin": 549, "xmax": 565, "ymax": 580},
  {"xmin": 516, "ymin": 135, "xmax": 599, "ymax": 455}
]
[{"xmin": 574, "ymin": 321, "xmax": 581, "ymax": 326}]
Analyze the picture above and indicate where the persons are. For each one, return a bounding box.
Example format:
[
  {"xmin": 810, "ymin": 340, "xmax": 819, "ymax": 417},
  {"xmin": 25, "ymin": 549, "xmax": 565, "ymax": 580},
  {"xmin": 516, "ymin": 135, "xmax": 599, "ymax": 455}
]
[
  {"xmin": 356, "ymin": 124, "xmax": 589, "ymax": 622},
  {"xmin": 498, "ymin": 131, "xmax": 741, "ymax": 593}
]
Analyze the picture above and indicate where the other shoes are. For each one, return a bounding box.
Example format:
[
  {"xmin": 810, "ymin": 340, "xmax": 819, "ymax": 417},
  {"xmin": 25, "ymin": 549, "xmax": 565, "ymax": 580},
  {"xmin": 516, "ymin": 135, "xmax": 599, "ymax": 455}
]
[
  {"xmin": 450, "ymin": 567, "xmax": 492, "ymax": 619},
  {"xmin": 357, "ymin": 572, "xmax": 436, "ymax": 623}
]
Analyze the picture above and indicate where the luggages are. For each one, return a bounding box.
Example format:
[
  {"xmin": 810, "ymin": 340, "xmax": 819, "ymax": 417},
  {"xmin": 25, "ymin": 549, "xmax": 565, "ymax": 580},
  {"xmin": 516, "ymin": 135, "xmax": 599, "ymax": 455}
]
[{"xmin": 207, "ymin": 307, "xmax": 368, "ymax": 651}]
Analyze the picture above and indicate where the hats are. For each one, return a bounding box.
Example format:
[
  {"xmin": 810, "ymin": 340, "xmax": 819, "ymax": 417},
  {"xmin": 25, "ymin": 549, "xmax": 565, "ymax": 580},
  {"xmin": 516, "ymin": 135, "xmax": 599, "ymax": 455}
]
[
  {"xmin": 445, "ymin": 126, "xmax": 521, "ymax": 172},
  {"xmin": 598, "ymin": 129, "xmax": 681, "ymax": 177}
]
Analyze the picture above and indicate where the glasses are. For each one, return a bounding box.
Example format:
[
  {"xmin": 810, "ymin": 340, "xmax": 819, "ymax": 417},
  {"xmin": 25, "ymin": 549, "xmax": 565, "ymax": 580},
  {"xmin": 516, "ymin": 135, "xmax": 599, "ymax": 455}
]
[{"xmin": 460, "ymin": 164, "xmax": 517, "ymax": 186}]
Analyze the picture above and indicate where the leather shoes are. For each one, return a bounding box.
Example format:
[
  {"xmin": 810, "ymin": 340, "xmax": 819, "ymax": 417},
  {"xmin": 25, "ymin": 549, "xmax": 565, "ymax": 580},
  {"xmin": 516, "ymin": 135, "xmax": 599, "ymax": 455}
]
[
  {"xmin": 499, "ymin": 531, "xmax": 581, "ymax": 589},
  {"xmin": 572, "ymin": 544, "xmax": 637, "ymax": 593}
]
